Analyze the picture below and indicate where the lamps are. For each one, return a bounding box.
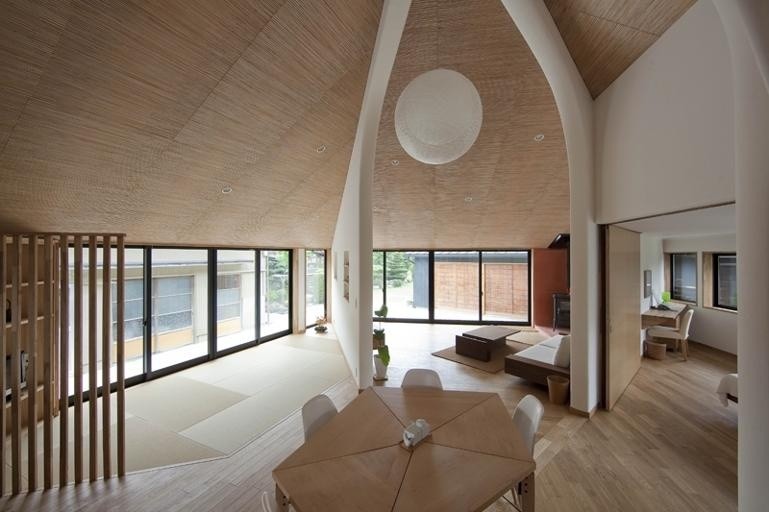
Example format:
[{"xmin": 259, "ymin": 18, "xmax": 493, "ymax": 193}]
[{"xmin": 393, "ymin": 0, "xmax": 484, "ymax": 165}]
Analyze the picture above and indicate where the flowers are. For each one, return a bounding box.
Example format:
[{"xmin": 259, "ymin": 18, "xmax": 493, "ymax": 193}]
[{"xmin": 314, "ymin": 317, "xmax": 328, "ymax": 326}]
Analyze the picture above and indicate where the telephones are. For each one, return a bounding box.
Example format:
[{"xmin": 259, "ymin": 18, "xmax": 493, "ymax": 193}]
[{"xmin": 658, "ymin": 304, "xmax": 671, "ymax": 310}]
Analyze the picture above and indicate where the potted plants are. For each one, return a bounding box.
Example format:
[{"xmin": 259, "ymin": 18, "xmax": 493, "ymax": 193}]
[
  {"xmin": 373, "ymin": 345, "xmax": 390, "ymax": 381},
  {"xmin": 373, "ymin": 328, "xmax": 385, "ymax": 349}
]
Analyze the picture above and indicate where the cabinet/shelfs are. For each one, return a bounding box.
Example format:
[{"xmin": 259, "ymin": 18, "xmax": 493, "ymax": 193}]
[
  {"xmin": 0, "ymin": 234, "xmax": 61, "ymax": 440},
  {"xmin": 343, "ymin": 255, "xmax": 349, "ymax": 303}
]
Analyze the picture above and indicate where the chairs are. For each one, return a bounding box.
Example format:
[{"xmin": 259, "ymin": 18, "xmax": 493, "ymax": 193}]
[
  {"xmin": 507, "ymin": 394, "xmax": 544, "ymax": 459},
  {"xmin": 262, "ymin": 492, "xmax": 271, "ymax": 512},
  {"xmin": 648, "ymin": 309, "xmax": 694, "ymax": 361},
  {"xmin": 301, "ymin": 395, "xmax": 340, "ymax": 444},
  {"xmin": 401, "ymin": 368, "xmax": 442, "ymax": 388}
]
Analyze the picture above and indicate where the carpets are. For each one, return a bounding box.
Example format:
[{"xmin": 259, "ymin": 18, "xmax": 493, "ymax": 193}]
[{"xmin": 432, "ymin": 339, "xmax": 533, "ymax": 374}]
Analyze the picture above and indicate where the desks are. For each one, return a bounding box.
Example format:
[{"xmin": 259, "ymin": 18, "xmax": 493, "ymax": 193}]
[
  {"xmin": 271, "ymin": 385, "xmax": 536, "ymax": 512},
  {"xmin": 642, "ymin": 302, "xmax": 688, "ymax": 352}
]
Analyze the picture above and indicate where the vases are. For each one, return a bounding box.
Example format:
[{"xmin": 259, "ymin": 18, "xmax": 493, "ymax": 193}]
[{"xmin": 314, "ymin": 326, "xmax": 328, "ymax": 334}]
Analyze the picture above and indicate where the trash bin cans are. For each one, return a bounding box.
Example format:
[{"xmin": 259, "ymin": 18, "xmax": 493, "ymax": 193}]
[{"xmin": 547, "ymin": 375, "xmax": 569, "ymax": 405}]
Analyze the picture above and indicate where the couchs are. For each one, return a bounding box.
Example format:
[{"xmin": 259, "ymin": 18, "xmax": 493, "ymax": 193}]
[{"xmin": 506, "ymin": 333, "xmax": 570, "ymax": 390}]
